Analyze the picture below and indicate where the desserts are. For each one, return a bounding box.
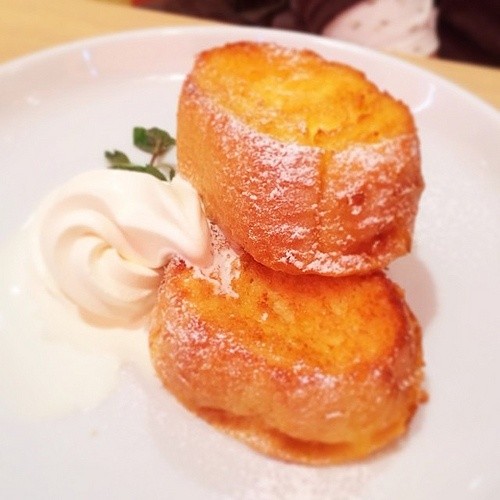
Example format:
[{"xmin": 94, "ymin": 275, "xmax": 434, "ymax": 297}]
[{"xmin": 40, "ymin": 38, "xmax": 427, "ymax": 469}]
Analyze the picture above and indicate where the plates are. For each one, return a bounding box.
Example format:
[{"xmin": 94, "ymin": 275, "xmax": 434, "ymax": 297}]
[{"xmin": 3, "ymin": 25, "xmax": 498, "ymax": 498}]
